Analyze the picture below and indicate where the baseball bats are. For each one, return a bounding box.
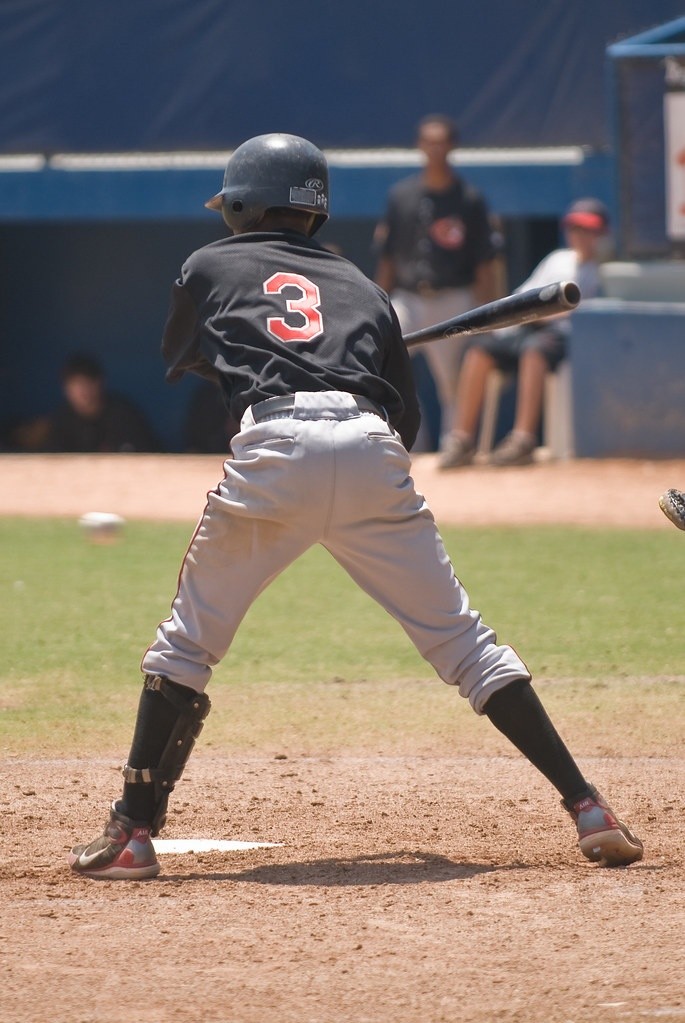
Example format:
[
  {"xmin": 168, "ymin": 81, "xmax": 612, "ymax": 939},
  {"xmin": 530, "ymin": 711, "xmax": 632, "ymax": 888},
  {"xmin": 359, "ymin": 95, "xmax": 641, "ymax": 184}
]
[{"xmin": 400, "ymin": 279, "xmax": 583, "ymax": 348}]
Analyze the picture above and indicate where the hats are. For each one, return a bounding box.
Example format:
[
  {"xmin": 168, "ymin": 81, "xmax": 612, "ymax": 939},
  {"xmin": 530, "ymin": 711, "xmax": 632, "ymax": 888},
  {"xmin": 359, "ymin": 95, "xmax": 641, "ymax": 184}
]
[{"xmin": 561, "ymin": 198, "xmax": 609, "ymax": 230}]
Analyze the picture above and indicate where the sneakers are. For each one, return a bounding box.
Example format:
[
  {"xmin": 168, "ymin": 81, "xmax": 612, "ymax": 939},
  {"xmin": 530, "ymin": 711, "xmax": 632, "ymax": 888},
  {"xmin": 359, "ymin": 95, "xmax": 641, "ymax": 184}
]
[
  {"xmin": 561, "ymin": 780, "xmax": 644, "ymax": 865},
  {"xmin": 68, "ymin": 798, "xmax": 161, "ymax": 879}
]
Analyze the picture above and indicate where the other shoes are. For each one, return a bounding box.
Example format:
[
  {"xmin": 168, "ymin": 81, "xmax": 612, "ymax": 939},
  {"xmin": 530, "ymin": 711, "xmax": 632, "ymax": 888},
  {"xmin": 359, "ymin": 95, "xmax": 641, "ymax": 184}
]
[
  {"xmin": 432, "ymin": 432, "xmax": 476, "ymax": 469},
  {"xmin": 659, "ymin": 489, "xmax": 685, "ymax": 531},
  {"xmin": 487, "ymin": 431, "xmax": 538, "ymax": 465}
]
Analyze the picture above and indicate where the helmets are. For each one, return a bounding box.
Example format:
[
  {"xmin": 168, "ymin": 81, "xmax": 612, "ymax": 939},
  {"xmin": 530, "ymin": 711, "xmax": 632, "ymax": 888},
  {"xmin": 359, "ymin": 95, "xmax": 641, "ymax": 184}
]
[{"xmin": 204, "ymin": 132, "xmax": 329, "ymax": 237}]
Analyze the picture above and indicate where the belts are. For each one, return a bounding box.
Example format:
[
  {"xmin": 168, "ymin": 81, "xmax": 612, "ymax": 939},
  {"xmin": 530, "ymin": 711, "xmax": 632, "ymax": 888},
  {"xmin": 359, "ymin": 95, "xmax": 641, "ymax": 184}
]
[{"xmin": 251, "ymin": 395, "xmax": 387, "ymax": 422}]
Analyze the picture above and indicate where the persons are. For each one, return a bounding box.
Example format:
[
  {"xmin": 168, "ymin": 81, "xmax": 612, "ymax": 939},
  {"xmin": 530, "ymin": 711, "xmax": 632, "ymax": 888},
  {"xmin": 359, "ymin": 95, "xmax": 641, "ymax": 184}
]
[
  {"xmin": 69, "ymin": 133, "xmax": 644, "ymax": 880},
  {"xmin": 372, "ymin": 114, "xmax": 493, "ymax": 452},
  {"xmin": 43, "ymin": 350, "xmax": 159, "ymax": 453},
  {"xmin": 435, "ymin": 198, "xmax": 608, "ymax": 468}
]
